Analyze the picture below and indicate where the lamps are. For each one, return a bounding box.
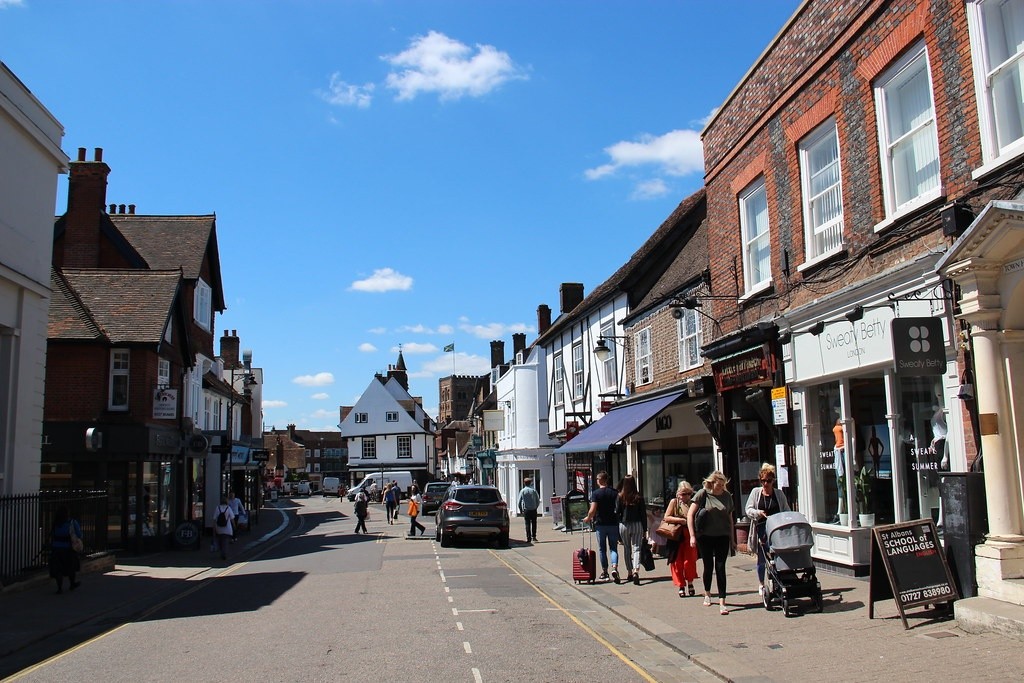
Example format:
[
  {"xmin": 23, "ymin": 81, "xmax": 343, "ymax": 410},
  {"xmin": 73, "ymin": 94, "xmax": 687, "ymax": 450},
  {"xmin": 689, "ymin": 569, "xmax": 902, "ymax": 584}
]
[
  {"xmin": 593, "ymin": 331, "xmax": 631, "ymax": 361},
  {"xmin": 668, "ymin": 302, "xmax": 703, "ymax": 320},
  {"xmin": 776, "ymin": 331, "xmax": 811, "ymax": 345},
  {"xmin": 809, "ymin": 319, "xmax": 854, "ymax": 336},
  {"xmin": 684, "ymin": 294, "xmax": 739, "ymax": 310},
  {"xmin": 686, "ymin": 379, "xmax": 704, "ymax": 398},
  {"xmin": 845, "ymin": 303, "xmax": 895, "ymax": 321}
]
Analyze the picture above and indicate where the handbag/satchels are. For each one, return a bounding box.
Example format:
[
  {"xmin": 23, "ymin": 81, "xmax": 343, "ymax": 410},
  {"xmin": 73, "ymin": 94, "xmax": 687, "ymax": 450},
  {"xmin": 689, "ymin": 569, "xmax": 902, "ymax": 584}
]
[
  {"xmin": 393, "ymin": 509, "xmax": 398, "ymax": 518},
  {"xmin": 69, "ymin": 519, "xmax": 83, "ymax": 551},
  {"xmin": 639, "ymin": 536, "xmax": 655, "ymax": 571},
  {"xmin": 209, "ymin": 544, "xmax": 218, "ymax": 551},
  {"xmin": 656, "ymin": 498, "xmax": 683, "ymax": 540}
]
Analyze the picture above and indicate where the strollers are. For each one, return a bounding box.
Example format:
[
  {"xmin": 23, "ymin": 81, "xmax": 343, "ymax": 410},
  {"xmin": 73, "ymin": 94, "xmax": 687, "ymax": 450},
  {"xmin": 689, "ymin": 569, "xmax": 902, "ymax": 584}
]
[{"xmin": 753, "ymin": 511, "xmax": 823, "ymax": 617}]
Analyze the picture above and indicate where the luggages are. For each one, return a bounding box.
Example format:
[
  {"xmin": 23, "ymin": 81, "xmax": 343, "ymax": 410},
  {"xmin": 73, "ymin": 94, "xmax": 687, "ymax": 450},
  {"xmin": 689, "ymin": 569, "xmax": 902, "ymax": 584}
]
[{"xmin": 572, "ymin": 519, "xmax": 596, "ymax": 585}]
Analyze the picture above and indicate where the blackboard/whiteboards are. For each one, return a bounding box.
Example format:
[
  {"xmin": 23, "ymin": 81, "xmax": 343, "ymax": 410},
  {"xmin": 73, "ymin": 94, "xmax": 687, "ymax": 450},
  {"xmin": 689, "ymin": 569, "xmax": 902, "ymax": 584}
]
[
  {"xmin": 869, "ymin": 518, "xmax": 957, "ymax": 610},
  {"xmin": 173, "ymin": 519, "xmax": 201, "ymax": 547}
]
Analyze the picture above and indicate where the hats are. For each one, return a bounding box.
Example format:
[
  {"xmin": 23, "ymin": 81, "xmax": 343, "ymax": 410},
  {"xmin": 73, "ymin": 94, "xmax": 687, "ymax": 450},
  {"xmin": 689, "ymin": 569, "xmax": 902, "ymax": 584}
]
[{"xmin": 524, "ymin": 478, "xmax": 531, "ymax": 481}]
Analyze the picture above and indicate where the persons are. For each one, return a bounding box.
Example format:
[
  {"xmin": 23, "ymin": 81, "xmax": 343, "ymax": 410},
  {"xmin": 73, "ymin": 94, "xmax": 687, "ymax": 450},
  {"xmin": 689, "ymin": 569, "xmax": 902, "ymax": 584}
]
[
  {"xmin": 226, "ymin": 491, "xmax": 246, "ymax": 543},
  {"xmin": 832, "ymin": 399, "xmax": 859, "ymax": 524},
  {"xmin": 354, "ymin": 485, "xmax": 370, "ymax": 534},
  {"xmin": 583, "ymin": 471, "xmax": 647, "ymax": 585},
  {"xmin": 214, "ymin": 494, "xmax": 236, "ymax": 559},
  {"xmin": 932, "ymin": 382, "xmax": 951, "ymax": 529},
  {"xmin": 52, "ymin": 504, "xmax": 83, "ymax": 593},
  {"xmin": 382, "ymin": 479, "xmax": 401, "ymax": 525},
  {"xmin": 745, "ymin": 462, "xmax": 792, "ymax": 596},
  {"xmin": 408, "ymin": 485, "xmax": 425, "ymax": 536},
  {"xmin": 687, "ymin": 471, "xmax": 736, "ymax": 615},
  {"xmin": 451, "ymin": 475, "xmax": 473, "ymax": 486},
  {"xmin": 518, "ymin": 477, "xmax": 540, "ymax": 542},
  {"xmin": 663, "ymin": 481, "xmax": 698, "ymax": 598}
]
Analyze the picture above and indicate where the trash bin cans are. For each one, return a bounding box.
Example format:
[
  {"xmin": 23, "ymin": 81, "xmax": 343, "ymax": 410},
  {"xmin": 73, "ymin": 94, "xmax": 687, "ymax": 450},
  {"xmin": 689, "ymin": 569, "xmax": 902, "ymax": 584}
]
[
  {"xmin": 376, "ymin": 490, "xmax": 382, "ymax": 502},
  {"xmin": 271, "ymin": 490, "xmax": 278, "ymax": 503}
]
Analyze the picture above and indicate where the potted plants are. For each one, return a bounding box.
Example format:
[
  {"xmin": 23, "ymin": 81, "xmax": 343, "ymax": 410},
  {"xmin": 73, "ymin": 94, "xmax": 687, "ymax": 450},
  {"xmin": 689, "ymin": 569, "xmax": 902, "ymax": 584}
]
[
  {"xmin": 837, "ymin": 472, "xmax": 848, "ymax": 526},
  {"xmin": 855, "ymin": 462, "xmax": 877, "ymax": 528}
]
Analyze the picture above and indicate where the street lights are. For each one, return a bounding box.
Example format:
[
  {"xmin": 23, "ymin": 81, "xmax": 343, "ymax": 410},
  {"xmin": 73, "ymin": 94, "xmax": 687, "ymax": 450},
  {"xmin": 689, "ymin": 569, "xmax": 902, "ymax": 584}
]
[
  {"xmin": 545, "ymin": 453, "xmax": 558, "ymax": 526},
  {"xmin": 469, "ymin": 417, "xmax": 496, "ymax": 487},
  {"xmin": 230, "ymin": 364, "xmax": 258, "ymax": 492}
]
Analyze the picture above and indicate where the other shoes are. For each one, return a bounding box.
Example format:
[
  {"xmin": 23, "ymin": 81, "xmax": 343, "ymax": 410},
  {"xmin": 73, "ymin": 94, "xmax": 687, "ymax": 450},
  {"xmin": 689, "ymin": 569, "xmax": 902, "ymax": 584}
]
[
  {"xmin": 533, "ymin": 537, "xmax": 538, "ymax": 541},
  {"xmin": 408, "ymin": 533, "xmax": 415, "ymax": 536},
  {"xmin": 703, "ymin": 595, "xmax": 711, "ymax": 605},
  {"xmin": 627, "ymin": 574, "xmax": 633, "ymax": 581},
  {"xmin": 421, "ymin": 527, "xmax": 425, "ymax": 535},
  {"xmin": 679, "ymin": 588, "xmax": 685, "ymax": 597},
  {"xmin": 688, "ymin": 584, "xmax": 695, "ymax": 596},
  {"xmin": 526, "ymin": 540, "xmax": 531, "ymax": 543},
  {"xmin": 719, "ymin": 606, "xmax": 728, "ymax": 615},
  {"xmin": 69, "ymin": 581, "xmax": 81, "ymax": 590},
  {"xmin": 633, "ymin": 572, "xmax": 639, "ymax": 584},
  {"xmin": 759, "ymin": 585, "xmax": 764, "ymax": 595}
]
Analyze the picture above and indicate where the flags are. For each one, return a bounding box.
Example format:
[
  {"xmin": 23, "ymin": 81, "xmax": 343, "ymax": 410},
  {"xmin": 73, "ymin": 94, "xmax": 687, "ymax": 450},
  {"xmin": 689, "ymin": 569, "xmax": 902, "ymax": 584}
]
[{"xmin": 444, "ymin": 343, "xmax": 454, "ymax": 352}]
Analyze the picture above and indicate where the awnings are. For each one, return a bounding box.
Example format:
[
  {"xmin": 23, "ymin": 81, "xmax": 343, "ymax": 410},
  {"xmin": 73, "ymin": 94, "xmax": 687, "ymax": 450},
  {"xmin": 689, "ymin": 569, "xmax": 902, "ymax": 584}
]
[{"xmin": 554, "ymin": 392, "xmax": 683, "ymax": 454}]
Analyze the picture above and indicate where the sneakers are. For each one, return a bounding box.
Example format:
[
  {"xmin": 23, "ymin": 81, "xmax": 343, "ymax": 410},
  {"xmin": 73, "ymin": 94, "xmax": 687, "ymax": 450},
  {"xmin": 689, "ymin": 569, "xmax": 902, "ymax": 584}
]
[
  {"xmin": 612, "ymin": 570, "xmax": 620, "ymax": 584},
  {"xmin": 598, "ymin": 573, "xmax": 610, "ymax": 581}
]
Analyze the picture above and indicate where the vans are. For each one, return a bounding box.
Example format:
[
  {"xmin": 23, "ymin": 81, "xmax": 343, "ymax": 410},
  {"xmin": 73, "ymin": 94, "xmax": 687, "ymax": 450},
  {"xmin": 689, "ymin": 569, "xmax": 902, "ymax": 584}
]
[
  {"xmin": 323, "ymin": 477, "xmax": 340, "ymax": 498},
  {"xmin": 347, "ymin": 471, "xmax": 413, "ymax": 502}
]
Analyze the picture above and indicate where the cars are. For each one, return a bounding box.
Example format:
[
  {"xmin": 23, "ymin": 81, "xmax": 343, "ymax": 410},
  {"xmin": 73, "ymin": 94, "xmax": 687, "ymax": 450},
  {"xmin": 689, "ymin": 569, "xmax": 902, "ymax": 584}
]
[{"xmin": 314, "ymin": 488, "xmax": 349, "ymax": 496}]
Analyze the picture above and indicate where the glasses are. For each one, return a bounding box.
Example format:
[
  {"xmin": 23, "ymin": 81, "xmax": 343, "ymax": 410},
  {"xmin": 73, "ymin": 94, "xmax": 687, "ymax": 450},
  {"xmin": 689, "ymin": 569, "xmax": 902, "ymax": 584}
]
[
  {"xmin": 760, "ymin": 478, "xmax": 774, "ymax": 483},
  {"xmin": 680, "ymin": 492, "xmax": 692, "ymax": 495}
]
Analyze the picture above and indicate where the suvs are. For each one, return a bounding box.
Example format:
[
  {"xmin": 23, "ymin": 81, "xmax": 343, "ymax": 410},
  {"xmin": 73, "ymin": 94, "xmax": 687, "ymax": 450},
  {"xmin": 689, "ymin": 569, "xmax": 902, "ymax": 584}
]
[
  {"xmin": 297, "ymin": 484, "xmax": 310, "ymax": 496},
  {"xmin": 434, "ymin": 485, "xmax": 510, "ymax": 547},
  {"xmin": 421, "ymin": 482, "xmax": 452, "ymax": 516}
]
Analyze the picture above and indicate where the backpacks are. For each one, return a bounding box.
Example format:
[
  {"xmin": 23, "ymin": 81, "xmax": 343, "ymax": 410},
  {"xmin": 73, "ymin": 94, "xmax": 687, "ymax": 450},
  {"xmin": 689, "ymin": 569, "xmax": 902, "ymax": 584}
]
[{"xmin": 217, "ymin": 505, "xmax": 230, "ymax": 527}]
